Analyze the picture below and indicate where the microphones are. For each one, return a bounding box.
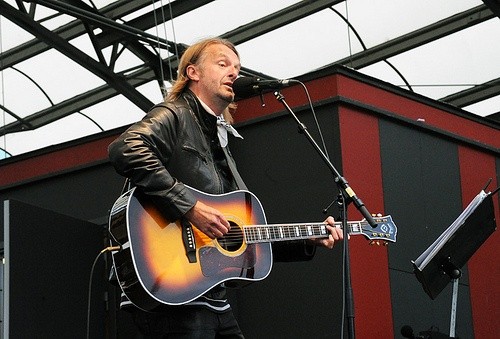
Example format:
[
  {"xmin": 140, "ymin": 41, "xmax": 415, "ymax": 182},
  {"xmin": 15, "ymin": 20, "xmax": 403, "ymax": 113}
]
[{"xmin": 232, "ymin": 76, "xmax": 299, "ymax": 97}]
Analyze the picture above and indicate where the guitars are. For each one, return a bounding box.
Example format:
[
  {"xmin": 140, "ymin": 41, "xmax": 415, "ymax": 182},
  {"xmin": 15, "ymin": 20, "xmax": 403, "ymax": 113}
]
[{"xmin": 108, "ymin": 178, "xmax": 397, "ymax": 313}]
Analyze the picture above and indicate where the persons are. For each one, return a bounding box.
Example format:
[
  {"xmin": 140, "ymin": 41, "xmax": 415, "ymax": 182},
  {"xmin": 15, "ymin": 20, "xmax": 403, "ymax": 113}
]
[{"xmin": 107, "ymin": 38, "xmax": 350, "ymax": 339}]
